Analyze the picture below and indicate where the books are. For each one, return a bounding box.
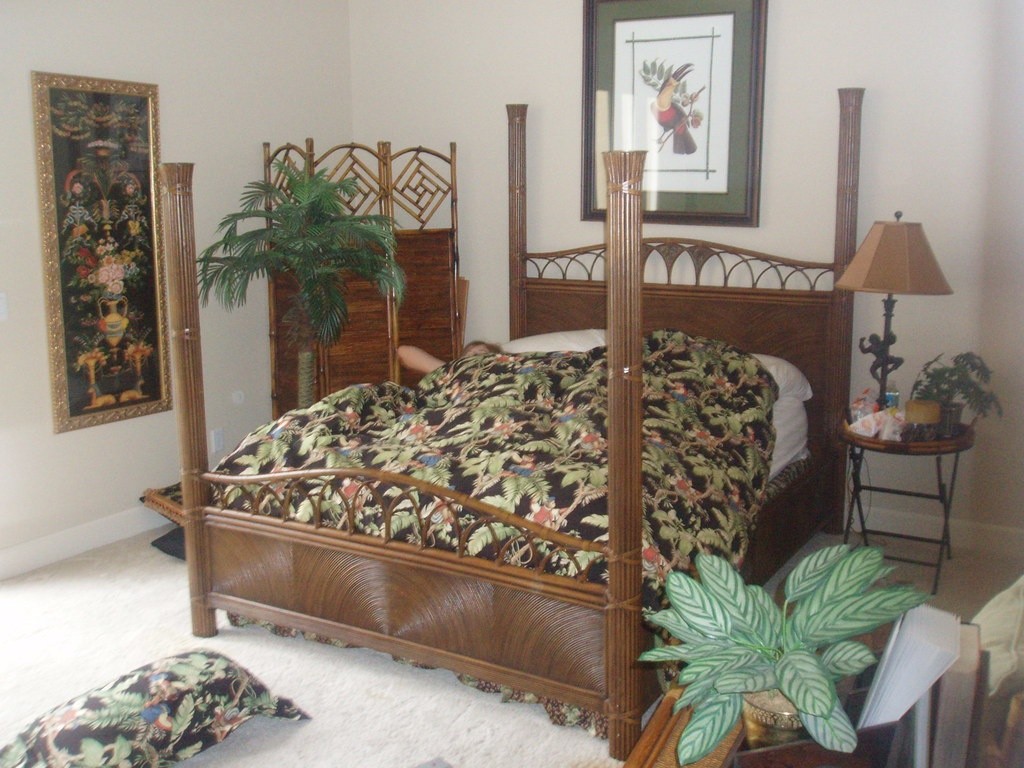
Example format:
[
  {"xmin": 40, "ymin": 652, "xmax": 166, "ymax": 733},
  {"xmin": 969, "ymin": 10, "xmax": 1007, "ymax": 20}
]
[{"xmin": 856, "ymin": 606, "xmax": 983, "ymax": 768}]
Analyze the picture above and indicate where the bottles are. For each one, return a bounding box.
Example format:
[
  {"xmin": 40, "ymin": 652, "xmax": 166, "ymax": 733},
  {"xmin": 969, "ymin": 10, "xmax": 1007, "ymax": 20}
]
[{"xmin": 886, "ymin": 379, "xmax": 899, "ymax": 409}]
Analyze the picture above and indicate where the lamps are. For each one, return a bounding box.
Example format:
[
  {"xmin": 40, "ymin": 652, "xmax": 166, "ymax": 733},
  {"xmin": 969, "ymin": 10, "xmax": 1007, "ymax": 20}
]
[{"xmin": 834, "ymin": 211, "xmax": 955, "ymax": 416}]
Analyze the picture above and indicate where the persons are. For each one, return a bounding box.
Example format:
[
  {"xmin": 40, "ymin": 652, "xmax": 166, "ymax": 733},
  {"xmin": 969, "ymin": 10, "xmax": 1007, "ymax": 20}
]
[{"xmin": 396, "ymin": 341, "xmax": 503, "ymax": 373}]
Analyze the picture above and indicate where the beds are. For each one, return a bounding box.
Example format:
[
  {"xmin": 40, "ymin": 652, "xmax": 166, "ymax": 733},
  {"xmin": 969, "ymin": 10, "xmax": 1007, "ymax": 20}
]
[{"xmin": 140, "ymin": 237, "xmax": 855, "ymax": 761}]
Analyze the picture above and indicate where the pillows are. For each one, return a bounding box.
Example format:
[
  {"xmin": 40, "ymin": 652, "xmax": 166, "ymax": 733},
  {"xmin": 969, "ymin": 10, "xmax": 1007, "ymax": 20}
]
[
  {"xmin": 748, "ymin": 351, "xmax": 813, "ymax": 403},
  {"xmin": 972, "ymin": 573, "xmax": 1024, "ymax": 700},
  {"xmin": 0, "ymin": 646, "xmax": 309, "ymax": 768},
  {"xmin": 151, "ymin": 528, "xmax": 185, "ymax": 561},
  {"xmin": 502, "ymin": 327, "xmax": 606, "ymax": 357}
]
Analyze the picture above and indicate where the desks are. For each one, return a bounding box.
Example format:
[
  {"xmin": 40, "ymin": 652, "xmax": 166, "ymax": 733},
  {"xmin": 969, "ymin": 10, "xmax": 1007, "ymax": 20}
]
[{"xmin": 840, "ymin": 413, "xmax": 975, "ymax": 595}]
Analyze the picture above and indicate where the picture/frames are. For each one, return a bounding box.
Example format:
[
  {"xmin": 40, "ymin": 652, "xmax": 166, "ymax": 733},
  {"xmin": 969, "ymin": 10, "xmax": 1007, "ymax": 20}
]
[
  {"xmin": 32, "ymin": 70, "xmax": 172, "ymax": 433},
  {"xmin": 579, "ymin": 0, "xmax": 769, "ymax": 228}
]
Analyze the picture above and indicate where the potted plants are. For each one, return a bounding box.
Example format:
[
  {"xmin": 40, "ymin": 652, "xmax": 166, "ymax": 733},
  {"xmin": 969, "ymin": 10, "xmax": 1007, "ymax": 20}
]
[
  {"xmin": 910, "ymin": 352, "xmax": 1003, "ymax": 440},
  {"xmin": 634, "ymin": 542, "xmax": 928, "ymax": 766}
]
